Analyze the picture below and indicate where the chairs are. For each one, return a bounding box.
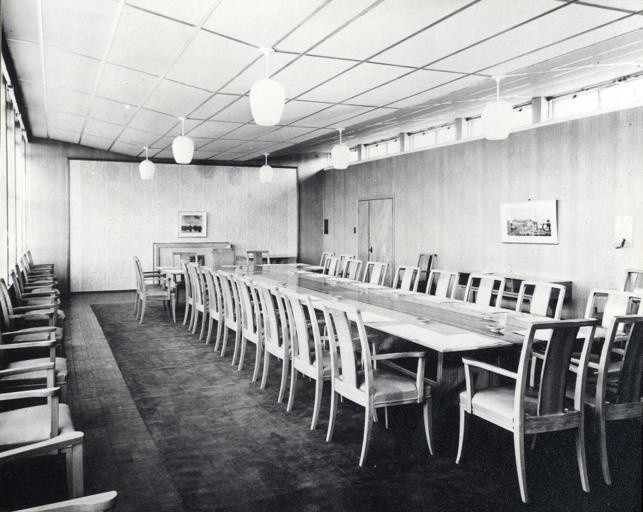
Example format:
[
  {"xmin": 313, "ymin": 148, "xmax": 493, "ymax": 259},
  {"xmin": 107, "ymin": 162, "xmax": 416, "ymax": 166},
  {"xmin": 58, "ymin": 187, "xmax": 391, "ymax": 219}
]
[
  {"xmin": 227, "ymin": 273, "xmax": 289, "ymax": 383},
  {"xmin": 199, "ymin": 266, "xmax": 235, "ymax": 352},
  {"xmin": 212, "ymin": 271, "xmax": 256, "ymax": 366},
  {"xmin": 456, "ymin": 318, "xmax": 599, "ymax": 504},
  {"xmin": 566, "ymin": 315, "xmax": 639, "ymax": 487},
  {"xmin": 276, "ymin": 293, "xmax": 379, "ymax": 432},
  {"xmin": 253, "ymin": 284, "xmax": 326, "ymax": 404},
  {"xmin": 128, "ymin": 249, "xmax": 641, "ymax": 332},
  {"xmin": 320, "ymin": 304, "xmax": 436, "ymax": 468},
  {"xmin": 0, "ymin": 250, "xmax": 119, "ymax": 512}
]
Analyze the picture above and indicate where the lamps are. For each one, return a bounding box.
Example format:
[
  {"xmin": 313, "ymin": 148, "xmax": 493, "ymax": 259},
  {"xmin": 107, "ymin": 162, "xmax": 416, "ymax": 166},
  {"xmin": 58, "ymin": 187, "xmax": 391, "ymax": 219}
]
[
  {"xmin": 479, "ymin": 75, "xmax": 514, "ymax": 141},
  {"xmin": 171, "ymin": 116, "xmax": 194, "ymax": 164},
  {"xmin": 330, "ymin": 128, "xmax": 350, "ymax": 170},
  {"xmin": 258, "ymin": 152, "xmax": 273, "ymax": 183},
  {"xmin": 249, "ymin": 47, "xmax": 284, "ymax": 128},
  {"xmin": 138, "ymin": 147, "xmax": 156, "ymax": 180}
]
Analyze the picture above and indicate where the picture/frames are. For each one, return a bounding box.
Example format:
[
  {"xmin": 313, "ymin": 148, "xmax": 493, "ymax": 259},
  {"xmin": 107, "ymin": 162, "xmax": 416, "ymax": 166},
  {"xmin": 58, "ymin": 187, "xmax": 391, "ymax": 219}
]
[
  {"xmin": 499, "ymin": 201, "xmax": 557, "ymax": 245},
  {"xmin": 178, "ymin": 211, "xmax": 208, "ymax": 238}
]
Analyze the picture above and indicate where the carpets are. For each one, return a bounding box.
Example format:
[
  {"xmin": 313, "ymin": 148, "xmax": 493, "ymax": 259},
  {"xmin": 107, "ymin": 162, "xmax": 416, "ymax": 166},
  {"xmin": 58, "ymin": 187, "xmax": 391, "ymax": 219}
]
[{"xmin": 94, "ymin": 301, "xmax": 641, "ymax": 511}]
